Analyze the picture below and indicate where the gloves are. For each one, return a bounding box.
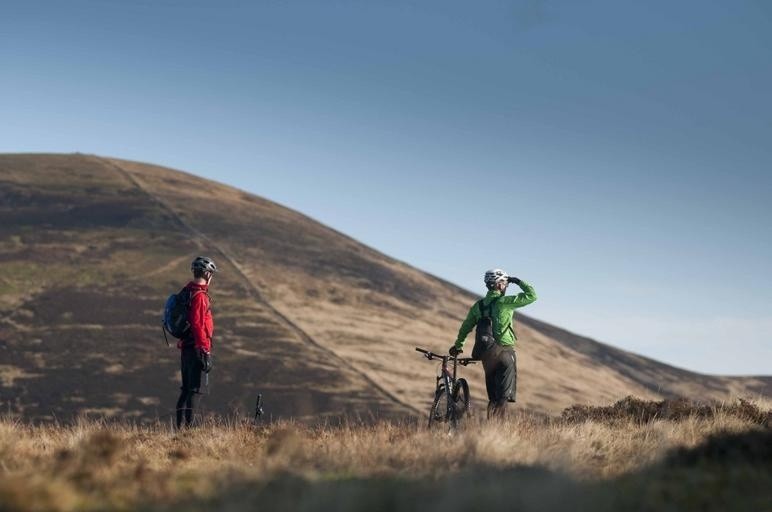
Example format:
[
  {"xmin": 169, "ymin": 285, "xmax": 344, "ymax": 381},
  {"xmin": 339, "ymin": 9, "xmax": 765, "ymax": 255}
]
[
  {"xmin": 449, "ymin": 346, "xmax": 463, "ymax": 356},
  {"xmin": 200, "ymin": 352, "xmax": 212, "ymax": 373},
  {"xmin": 508, "ymin": 277, "xmax": 521, "ymax": 286}
]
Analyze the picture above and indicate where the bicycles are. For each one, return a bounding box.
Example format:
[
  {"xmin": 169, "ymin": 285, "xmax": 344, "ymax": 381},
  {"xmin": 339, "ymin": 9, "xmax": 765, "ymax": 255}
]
[{"xmin": 416, "ymin": 346, "xmax": 480, "ymax": 430}]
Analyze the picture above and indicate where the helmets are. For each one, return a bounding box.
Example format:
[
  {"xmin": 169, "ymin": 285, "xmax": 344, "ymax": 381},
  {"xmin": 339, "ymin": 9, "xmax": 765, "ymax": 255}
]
[
  {"xmin": 191, "ymin": 257, "xmax": 216, "ymax": 272},
  {"xmin": 485, "ymin": 270, "xmax": 511, "ymax": 286}
]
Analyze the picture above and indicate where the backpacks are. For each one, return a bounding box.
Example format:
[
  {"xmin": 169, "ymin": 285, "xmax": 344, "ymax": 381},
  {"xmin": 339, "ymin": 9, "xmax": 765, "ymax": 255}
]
[
  {"xmin": 472, "ymin": 296, "xmax": 510, "ymax": 358},
  {"xmin": 162, "ymin": 287, "xmax": 204, "ymax": 345}
]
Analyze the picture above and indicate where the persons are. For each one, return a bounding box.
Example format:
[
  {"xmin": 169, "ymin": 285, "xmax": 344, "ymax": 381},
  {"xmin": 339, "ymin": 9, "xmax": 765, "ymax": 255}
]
[
  {"xmin": 448, "ymin": 268, "xmax": 537, "ymax": 420},
  {"xmin": 168, "ymin": 256, "xmax": 216, "ymax": 428}
]
